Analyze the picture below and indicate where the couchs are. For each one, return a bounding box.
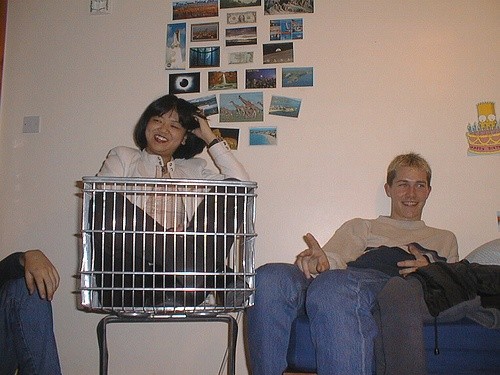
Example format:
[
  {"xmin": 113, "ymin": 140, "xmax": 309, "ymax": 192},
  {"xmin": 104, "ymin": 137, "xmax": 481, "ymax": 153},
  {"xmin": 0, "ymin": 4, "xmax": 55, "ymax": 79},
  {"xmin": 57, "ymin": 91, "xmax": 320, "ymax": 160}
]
[{"xmin": 242, "ymin": 310, "xmax": 500, "ymax": 375}]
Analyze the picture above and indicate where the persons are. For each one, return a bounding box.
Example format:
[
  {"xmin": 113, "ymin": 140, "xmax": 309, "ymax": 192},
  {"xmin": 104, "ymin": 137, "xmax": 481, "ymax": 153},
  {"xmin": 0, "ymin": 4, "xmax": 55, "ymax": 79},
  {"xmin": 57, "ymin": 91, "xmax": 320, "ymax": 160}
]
[
  {"xmin": 244, "ymin": 153, "xmax": 459, "ymax": 375},
  {"xmin": 371, "ymin": 231, "xmax": 500, "ymax": 375},
  {"xmin": 87, "ymin": 94, "xmax": 253, "ymax": 307},
  {"xmin": 0, "ymin": 249, "xmax": 62, "ymax": 375}
]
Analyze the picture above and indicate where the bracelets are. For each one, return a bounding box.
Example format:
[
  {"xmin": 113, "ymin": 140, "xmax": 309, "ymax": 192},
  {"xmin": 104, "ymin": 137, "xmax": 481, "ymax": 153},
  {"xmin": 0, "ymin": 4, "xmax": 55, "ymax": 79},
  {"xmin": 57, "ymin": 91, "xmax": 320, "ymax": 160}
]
[{"xmin": 208, "ymin": 137, "xmax": 223, "ymax": 148}]
